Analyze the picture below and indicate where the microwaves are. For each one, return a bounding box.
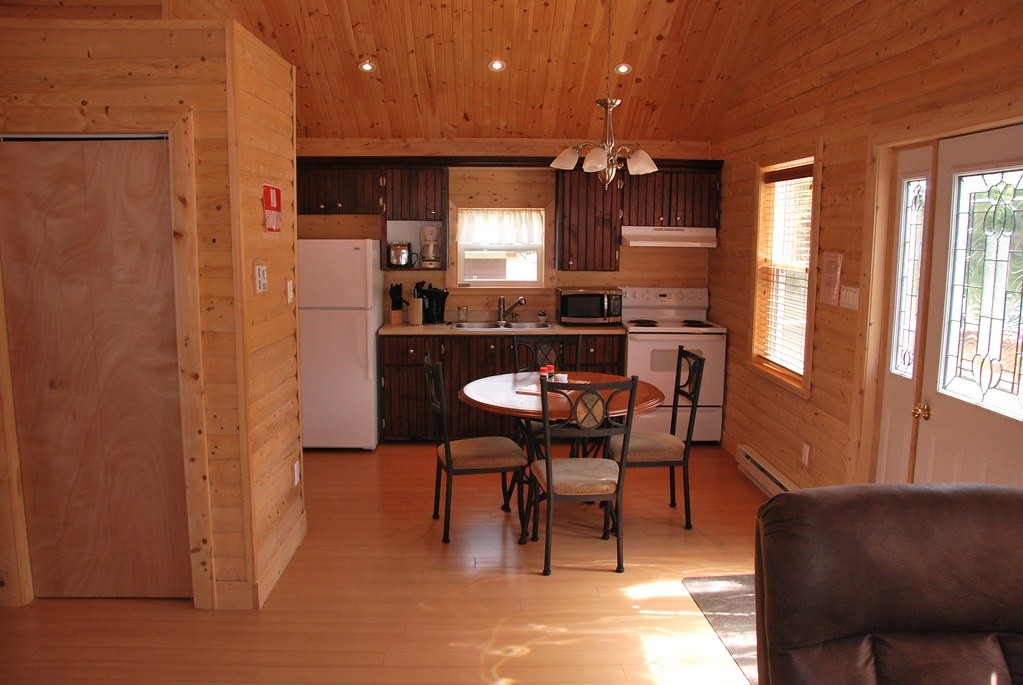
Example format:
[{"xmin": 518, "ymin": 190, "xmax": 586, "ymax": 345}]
[{"xmin": 555, "ymin": 286, "xmax": 623, "ymax": 325}]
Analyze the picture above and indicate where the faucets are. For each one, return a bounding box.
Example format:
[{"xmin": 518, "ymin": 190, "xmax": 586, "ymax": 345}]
[{"xmin": 498, "ymin": 296, "xmax": 526, "ymax": 321}]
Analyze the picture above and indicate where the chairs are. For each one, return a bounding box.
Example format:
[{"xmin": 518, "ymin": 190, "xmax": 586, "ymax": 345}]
[
  {"xmin": 511, "ymin": 333, "xmax": 582, "ymax": 458},
  {"xmin": 599, "ymin": 345, "xmax": 706, "ymax": 530},
  {"xmin": 754, "ymin": 483, "xmax": 1023, "ymax": 685},
  {"xmin": 530, "ymin": 375, "xmax": 639, "ymax": 576},
  {"xmin": 421, "ymin": 347, "xmax": 530, "ymax": 545}
]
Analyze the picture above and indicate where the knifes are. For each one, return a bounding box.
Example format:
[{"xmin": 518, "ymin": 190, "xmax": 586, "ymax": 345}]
[{"xmin": 388, "ymin": 283, "xmax": 403, "ymax": 310}]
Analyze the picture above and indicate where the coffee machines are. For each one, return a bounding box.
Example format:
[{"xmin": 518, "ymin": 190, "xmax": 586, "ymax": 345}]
[{"xmin": 413, "ymin": 281, "xmax": 449, "ymax": 325}]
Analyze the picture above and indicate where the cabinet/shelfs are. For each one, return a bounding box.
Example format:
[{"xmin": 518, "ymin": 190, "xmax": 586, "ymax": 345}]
[
  {"xmin": 296, "ymin": 156, "xmax": 380, "ymax": 215},
  {"xmin": 380, "ymin": 155, "xmax": 450, "ymax": 272},
  {"xmin": 561, "ymin": 334, "xmax": 626, "ymax": 446},
  {"xmin": 377, "ymin": 336, "xmax": 452, "ymax": 445},
  {"xmin": 451, "ymin": 334, "xmax": 562, "ymax": 445},
  {"xmin": 622, "ymin": 156, "xmax": 725, "ymax": 229},
  {"xmin": 553, "ymin": 156, "xmax": 622, "ymax": 272}
]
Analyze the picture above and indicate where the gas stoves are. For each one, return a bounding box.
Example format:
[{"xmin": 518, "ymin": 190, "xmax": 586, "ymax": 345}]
[{"xmin": 617, "ymin": 286, "xmax": 727, "ymax": 334}]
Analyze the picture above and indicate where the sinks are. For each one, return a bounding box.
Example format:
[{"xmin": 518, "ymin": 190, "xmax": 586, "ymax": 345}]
[
  {"xmin": 451, "ymin": 322, "xmax": 500, "ymax": 331},
  {"xmin": 504, "ymin": 322, "xmax": 551, "ymax": 330}
]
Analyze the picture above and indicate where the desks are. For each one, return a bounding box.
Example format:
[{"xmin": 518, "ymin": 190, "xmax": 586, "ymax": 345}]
[{"xmin": 456, "ymin": 371, "xmax": 666, "ymax": 545}]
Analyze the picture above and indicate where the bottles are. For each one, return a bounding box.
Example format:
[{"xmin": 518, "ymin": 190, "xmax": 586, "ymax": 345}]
[
  {"xmin": 457, "ymin": 305, "xmax": 467, "ymax": 321},
  {"xmin": 539, "ymin": 366, "xmax": 548, "ymax": 382},
  {"xmin": 546, "ymin": 365, "xmax": 554, "ymax": 378}
]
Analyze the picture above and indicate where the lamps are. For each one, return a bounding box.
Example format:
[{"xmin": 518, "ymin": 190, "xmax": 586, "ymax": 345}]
[{"xmin": 550, "ymin": 0, "xmax": 659, "ymax": 190}]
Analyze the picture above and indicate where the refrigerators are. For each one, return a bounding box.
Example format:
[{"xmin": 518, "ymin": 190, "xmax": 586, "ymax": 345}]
[{"xmin": 296, "ymin": 237, "xmax": 384, "ymax": 450}]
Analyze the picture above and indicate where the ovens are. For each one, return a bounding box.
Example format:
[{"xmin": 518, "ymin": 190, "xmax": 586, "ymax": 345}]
[{"xmin": 624, "ymin": 333, "xmax": 727, "ymax": 441}]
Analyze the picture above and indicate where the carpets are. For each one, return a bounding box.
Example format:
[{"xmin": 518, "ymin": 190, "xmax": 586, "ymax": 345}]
[{"xmin": 681, "ymin": 573, "xmax": 759, "ymax": 685}]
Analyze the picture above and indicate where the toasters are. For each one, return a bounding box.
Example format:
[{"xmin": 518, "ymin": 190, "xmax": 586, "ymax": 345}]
[{"xmin": 387, "ymin": 240, "xmax": 412, "ymax": 269}]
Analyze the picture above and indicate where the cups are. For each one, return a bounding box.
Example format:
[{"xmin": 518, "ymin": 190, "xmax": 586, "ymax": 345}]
[
  {"xmin": 554, "ymin": 380, "xmax": 568, "ymax": 393},
  {"xmin": 408, "ymin": 298, "xmax": 424, "ymax": 325}
]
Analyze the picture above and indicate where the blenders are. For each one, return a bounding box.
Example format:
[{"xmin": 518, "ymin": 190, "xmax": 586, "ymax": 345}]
[{"xmin": 419, "ymin": 225, "xmax": 442, "ymax": 268}]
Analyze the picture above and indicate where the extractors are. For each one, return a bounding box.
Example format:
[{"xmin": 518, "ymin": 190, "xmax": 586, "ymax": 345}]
[{"xmin": 620, "ymin": 226, "xmax": 717, "ymax": 249}]
